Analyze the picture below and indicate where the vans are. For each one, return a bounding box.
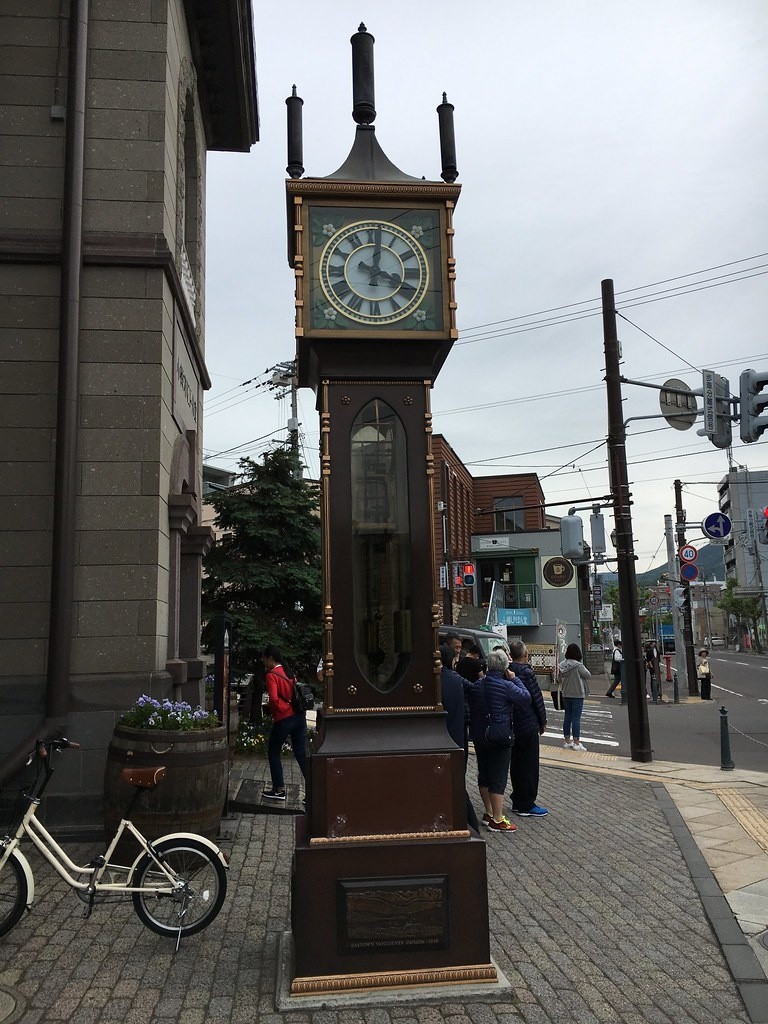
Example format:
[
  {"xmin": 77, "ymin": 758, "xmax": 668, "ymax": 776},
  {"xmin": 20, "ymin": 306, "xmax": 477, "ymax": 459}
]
[{"xmin": 704, "ymin": 637, "xmax": 724, "ymax": 646}]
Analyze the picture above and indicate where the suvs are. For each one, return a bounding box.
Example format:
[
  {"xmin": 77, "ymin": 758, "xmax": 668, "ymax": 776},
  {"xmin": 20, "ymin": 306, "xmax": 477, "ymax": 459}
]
[{"xmin": 438, "ymin": 624, "xmax": 511, "ymax": 667}]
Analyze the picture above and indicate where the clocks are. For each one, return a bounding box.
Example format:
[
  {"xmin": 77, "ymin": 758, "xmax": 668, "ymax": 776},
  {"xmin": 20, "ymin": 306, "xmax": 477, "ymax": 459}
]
[{"xmin": 320, "ymin": 218, "xmax": 430, "ymax": 323}]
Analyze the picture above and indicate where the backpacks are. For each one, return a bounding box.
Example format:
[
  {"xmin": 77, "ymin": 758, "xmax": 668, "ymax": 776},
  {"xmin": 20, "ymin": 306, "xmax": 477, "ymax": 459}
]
[{"xmin": 268, "ymin": 672, "xmax": 314, "ymax": 711}]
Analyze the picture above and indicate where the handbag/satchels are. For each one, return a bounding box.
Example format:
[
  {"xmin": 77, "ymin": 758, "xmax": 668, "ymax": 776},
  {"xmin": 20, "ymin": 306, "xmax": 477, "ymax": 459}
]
[
  {"xmin": 700, "ymin": 663, "xmax": 709, "ymax": 674},
  {"xmin": 488, "ymin": 718, "xmax": 514, "ymax": 747},
  {"xmin": 550, "ymin": 683, "xmax": 564, "ymax": 710},
  {"xmin": 611, "ymin": 661, "xmax": 621, "ymax": 675},
  {"xmin": 697, "ymin": 669, "xmax": 706, "ymax": 679}
]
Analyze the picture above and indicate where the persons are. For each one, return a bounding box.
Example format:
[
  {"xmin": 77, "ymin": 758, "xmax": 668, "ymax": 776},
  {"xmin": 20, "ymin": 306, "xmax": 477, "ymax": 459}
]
[
  {"xmin": 642, "ymin": 641, "xmax": 660, "ymax": 698},
  {"xmin": 468, "ymin": 650, "xmax": 531, "ymax": 832},
  {"xmin": 605, "ymin": 641, "xmax": 622, "ymax": 698},
  {"xmin": 439, "ymin": 644, "xmax": 475, "ymax": 764},
  {"xmin": 443, "ymin": 631, "xmax": 463, "ymax": 671},
  {"xmin": 262, "ymin": 645, "xmax": 310, "ymax": 804},
  {"xmin": 458, "ymin": 647, "xmax": 485, "ymax": 741},
  {"xmin": 696, "ymin": 648, "xmax": 713, "ymax": 700},
  {"xmin": 510, "ymin": 640, "xmax": 548, "ymax": 816},
  {"xmin": 558, "ymin": 643, "xmax": 591, "ymax": 751}
]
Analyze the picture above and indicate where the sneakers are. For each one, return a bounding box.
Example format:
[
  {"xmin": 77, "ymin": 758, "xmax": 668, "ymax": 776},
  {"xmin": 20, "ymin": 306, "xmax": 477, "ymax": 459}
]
[
  {"xmin": 573, "ymin": 743, "xmax": 587, "ymax": 751},
  {"xmin": 483, "ymin": 812, "xmax": 493, "ymax": 825},
  {"xmin": 487, "ymin": 816, "xmax": 517, "ymax": 833},
  {"xmin": 262, "ymin": 789, "xmax": 286, "ymax": 799},
  {"xmin": 513, "ymin": 805, "xmax": 548, "ymax": 817},
  {"xmin": 564, "ymin": 741, "xmax": 573, "ymax": 749}
]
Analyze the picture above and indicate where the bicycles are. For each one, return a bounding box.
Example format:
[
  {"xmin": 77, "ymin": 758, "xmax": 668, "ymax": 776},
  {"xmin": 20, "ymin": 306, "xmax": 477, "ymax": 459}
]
[{"xmin": 0, "ymin": 730, "xmax": 232, "ymax": 954}]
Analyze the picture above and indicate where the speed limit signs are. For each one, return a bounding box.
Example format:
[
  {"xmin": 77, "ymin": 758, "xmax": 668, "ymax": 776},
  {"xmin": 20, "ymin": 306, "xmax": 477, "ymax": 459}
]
[
  {"xmin": 650, "ymin": 596, "xmax": 659, "ymax": 605},
  {"xmin": 679, "ymin": 545, "xmax": 698, "ymax": 563}
]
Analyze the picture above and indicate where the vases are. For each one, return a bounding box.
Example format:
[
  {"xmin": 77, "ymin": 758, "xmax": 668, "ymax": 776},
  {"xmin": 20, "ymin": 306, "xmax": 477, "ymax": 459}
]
[{"xmin": 103, "ymin": 727, "xmax": 230, "ymax": 873}]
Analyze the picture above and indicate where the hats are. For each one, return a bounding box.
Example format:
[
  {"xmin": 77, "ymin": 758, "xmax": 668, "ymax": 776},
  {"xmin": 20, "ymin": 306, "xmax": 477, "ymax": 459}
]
[{"xmin": 698, "ymin": 648, "xmax": 709, "ymax": 656}]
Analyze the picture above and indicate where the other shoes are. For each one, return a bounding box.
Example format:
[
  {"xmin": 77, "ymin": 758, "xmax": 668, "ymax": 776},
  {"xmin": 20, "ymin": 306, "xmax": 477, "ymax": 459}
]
[
  {"xmin": 702, "ymin": 697, "xmax": 713, "ymax": 700},
  {"xmin": 606, "ymin": 693, "xmax": 615, "ymax": 698}
]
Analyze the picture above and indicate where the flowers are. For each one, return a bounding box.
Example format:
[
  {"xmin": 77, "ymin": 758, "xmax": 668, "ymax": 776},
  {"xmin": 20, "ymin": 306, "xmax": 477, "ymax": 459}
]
[{"xmin": 121, "ymin": 694, "xmax": 219, "ymax": 730}]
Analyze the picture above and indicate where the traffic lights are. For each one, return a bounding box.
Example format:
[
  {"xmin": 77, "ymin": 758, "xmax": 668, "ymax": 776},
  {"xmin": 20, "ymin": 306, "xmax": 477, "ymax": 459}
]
[
  {"xmin": 446, "ymin": 560, "xmax": 475, "ymax": 591},
  {"xmin": 738, "ymin": 368, "xmax": 768, "ymax": 443},
  {"xmin": 674, "ymin": 587, "xmax": 689, "ymax": 609},
  {"xmin": 757, "ymin": 506, "xmax": 768, "ymax": 545}
]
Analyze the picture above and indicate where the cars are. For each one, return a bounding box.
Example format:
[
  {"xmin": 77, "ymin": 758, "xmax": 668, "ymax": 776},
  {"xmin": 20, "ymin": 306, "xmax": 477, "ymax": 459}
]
[
  {"xmin": 588, "ymin": 643, "xmax": 613, "ymax": 656},
  {"xmin": 659, "ymin": 638, "xmax": 676, "ymax": 655}
]
